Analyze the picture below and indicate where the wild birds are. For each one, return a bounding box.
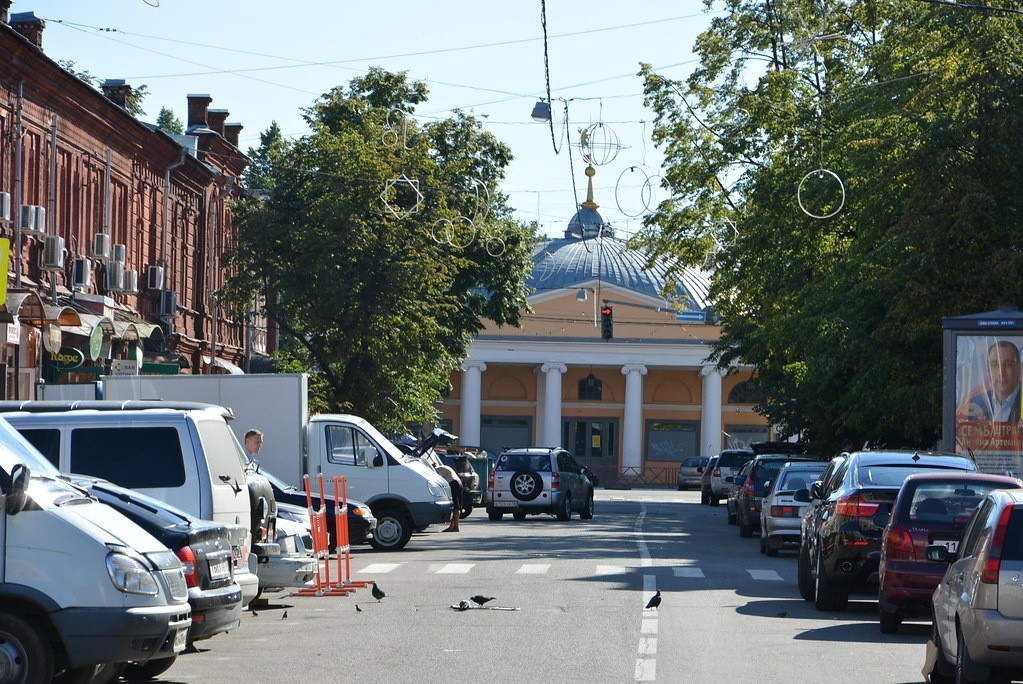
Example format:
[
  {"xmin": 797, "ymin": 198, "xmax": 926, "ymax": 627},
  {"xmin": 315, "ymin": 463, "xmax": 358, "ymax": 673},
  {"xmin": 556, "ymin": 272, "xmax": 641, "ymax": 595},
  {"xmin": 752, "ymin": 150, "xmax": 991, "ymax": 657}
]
[
  {"xmin": 459, "ymin": 600, "xmax": 469, "ymax": 610},
  {"xmin": 470, "ymin": 596, "xmax": 496, "ymax": 608},
  {"xmin": 372, "ymin": 583, "xmax": 388, "ymax": 602},
  {"xmin": 355, "ymin": 605, "xmax": 362, "ymax": 612},
  {"xmin": 778, "ymin": 611, "xmax": 786, "ymax": 617},
  {"xmin": 252, "ymin": 609, "xmax": 258, "ymax": 617},
  {"xmin": 282, "ymin": 611, "xmax": 288, "ymax": 620},
  {"xmin": 645, "ymin": 589, "xmax": 661, "ymax": 610}
]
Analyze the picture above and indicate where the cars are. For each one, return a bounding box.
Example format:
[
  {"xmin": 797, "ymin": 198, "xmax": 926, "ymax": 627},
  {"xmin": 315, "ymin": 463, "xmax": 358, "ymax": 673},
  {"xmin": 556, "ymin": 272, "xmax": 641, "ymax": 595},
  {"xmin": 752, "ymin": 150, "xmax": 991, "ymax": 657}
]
[
  {"xmin": 241, "ymin": 445, "xmax": 376, "ymax": 552},
  {"xmin": 929, "ymin": 488, "xmax": 1023, "ymax": 684},
  {"xmin": 759, "ymin": 461, "xmax": 830, "ymax": 557},
  {"xmin": 332, "ymin": 427, "xmax": 496, "ymax": 520},
  {"xmin": 677, "ymin": 456, "xmax": 714, "ymax": 491},
  {"xmin": 877, "ymin": 472, "xmax": 1023, "ymax": 632},
  {"xmin": 62, "ymin": 473, "xmax": 244, "ymax": 643},
  {"xmin": 248, "ymin": 501, "xmax": 329, "ymax": 589}
]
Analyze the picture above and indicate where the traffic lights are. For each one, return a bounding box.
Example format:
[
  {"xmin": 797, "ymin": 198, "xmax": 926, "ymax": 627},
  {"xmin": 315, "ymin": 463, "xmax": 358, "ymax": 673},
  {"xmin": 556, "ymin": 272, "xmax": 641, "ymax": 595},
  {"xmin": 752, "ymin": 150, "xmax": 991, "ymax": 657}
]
[{"xmin": 600, "ymin": 306, "xmax": 613, "ymax": 340}]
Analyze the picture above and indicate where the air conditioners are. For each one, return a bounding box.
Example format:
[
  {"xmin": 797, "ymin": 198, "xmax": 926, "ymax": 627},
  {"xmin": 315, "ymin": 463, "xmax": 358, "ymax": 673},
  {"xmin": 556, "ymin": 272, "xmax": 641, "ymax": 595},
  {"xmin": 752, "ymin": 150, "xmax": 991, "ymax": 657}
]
[
  {"xmin": 0, "ymin": 192, "xmax": 10, "ymax": 221},
  {"xmin": 147, "ymin": 266, "xmax": 164, "ymax": 290},
  {"xmin": 33, "ymin": 206, "xmax": 46, "ymax": 232},
  {"xmin": 164, "ymin": 291, "xmax": 176, "ymax": 316},
  {"xmin": 123, "ymin": 270, "xmax": 137, "ymax": 292},
  {"xmin": 113, "ymin": 244, "xmax": 125, "ymax": 265},
  {"xmin": 21, "ymin": 205, "xmax": 36, "ymax": 230},
  {"xmin": 44, "ymin": 236, "xmax": 64, "ymax": 268},
  {"xmin": 74, "ymin": 258, "xmax": 91, "ymax": 286},
  {"xmin": 110, "ymin": 261, "xmax": 123, "ymax": 288},
  {"xmin": 94, "ymin": 233, "xmax": 110, "ymax": 258}
]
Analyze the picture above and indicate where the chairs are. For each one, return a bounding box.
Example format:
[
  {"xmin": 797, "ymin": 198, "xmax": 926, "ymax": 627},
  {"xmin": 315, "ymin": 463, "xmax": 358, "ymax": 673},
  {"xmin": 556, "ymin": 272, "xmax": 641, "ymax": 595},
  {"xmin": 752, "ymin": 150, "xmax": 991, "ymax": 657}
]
[
  {"xmin": 537, "ymin": 459, "xmax": 547, "ymax": 470},
  {"xmin": 916, "ymin": 498, "xmax": 948, "ymax": 514},
  {"xmin": 787, "ymin": 478, "xmax": 807, "ymax": 491},
  {"xmin": 507, "ymin": 456, "xmax": 522, "ymax": 470},
  {"xmin": 691, "ymin": 461, "xmax": 698, "ymax": 465}
]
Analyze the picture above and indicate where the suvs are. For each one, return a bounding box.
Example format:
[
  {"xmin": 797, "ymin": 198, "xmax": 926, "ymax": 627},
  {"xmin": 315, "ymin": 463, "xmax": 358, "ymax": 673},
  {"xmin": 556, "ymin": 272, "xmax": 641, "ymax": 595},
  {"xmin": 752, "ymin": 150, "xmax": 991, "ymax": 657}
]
[
  {"xmin": 792, "ymin": 450, "xmax": 980, "ymax": 612},
  {"xmin": 486, "ymin": 445, "xmax": 594, "ymax": 521},
  {"xmin": 696, "ymin": 448, "xmax": 815, "ymax": 537}
]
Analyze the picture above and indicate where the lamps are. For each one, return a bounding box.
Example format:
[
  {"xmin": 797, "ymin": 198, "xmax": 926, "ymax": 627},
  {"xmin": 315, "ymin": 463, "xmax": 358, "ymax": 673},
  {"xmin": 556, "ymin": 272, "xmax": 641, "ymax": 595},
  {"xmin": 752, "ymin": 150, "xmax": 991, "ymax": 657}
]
[
  {"xmin": 576, "ymin": 289, "xmax": 588, "ymax": 301},
  {"xmin": 530, "ymin": 97, "xmax": 552, "ymax": 123}
]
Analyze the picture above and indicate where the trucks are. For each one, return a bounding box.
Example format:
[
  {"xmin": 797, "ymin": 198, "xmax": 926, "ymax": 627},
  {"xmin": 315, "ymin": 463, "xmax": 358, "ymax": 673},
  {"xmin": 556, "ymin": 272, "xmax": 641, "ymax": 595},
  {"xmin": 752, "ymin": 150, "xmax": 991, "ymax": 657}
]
[{"xmin": 33, "ymin": 373, "xmax": 455, "ymax": 552}]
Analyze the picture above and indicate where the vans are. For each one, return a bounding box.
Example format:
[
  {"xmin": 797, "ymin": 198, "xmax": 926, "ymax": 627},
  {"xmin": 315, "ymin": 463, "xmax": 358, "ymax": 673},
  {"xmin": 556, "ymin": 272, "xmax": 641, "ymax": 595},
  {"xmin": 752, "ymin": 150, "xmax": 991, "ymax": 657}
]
[
  {"xmin": 0, "ymin": 414, "xmax": 192, "ymax": 684},
  {"xmin": 0, "ymin": 408, "xmax": 260, "ymax": 609}
]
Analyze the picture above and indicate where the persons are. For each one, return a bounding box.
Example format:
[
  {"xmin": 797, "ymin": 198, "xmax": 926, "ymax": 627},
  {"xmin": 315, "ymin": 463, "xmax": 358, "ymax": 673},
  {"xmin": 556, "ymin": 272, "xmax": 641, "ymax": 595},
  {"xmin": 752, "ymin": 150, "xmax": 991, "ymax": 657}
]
[
  {"xmin": 111, "ymin": 353, "xmax": 121, "ymax": 370},
  {"xmin": 241, "ymin": 429, "xmax": 264, "ymax": 464},
  {"xmin": 968, "ymin": 341, "xmax": 1023, "ymax": 423},
  {"xmin": 432, "ymin": 462, "xmax": 463, "ymax": 532}
]
